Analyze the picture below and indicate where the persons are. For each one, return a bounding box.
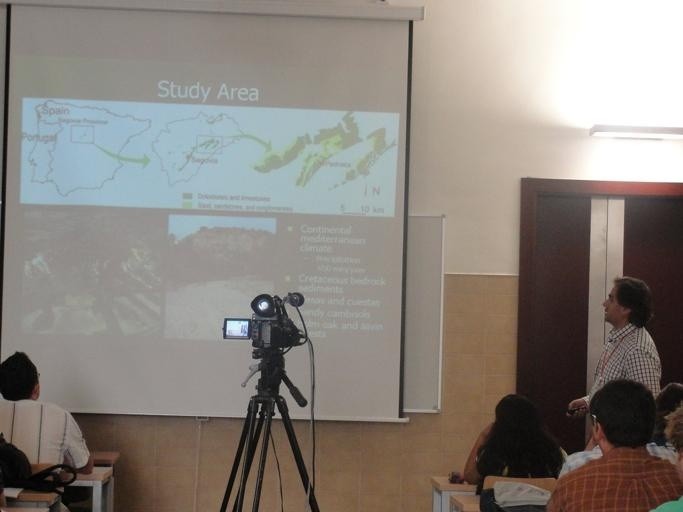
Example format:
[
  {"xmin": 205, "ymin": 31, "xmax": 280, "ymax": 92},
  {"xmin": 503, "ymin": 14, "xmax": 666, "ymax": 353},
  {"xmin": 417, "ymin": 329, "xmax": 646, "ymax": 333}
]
[
  {"xmin": 647, "ymin": 383, "xmax": 682, "ymax": 466},
  {"xmin": 543, "ymin": 376, "xmax": 682, "ymax": 512},
  {"xmin": 564, "ymin": 274, "xmax": 661, "ymax": 418},
  {"xmin": 651, "ymin": 405, "xmax": 683, "ymax": 511},
  {"xmin": 0, "ymin": 347, "xmax": 94, "ymax": 511},
  {"xmin": 462, "ymin": 391, "xmax": 569, "ymax": 511}
]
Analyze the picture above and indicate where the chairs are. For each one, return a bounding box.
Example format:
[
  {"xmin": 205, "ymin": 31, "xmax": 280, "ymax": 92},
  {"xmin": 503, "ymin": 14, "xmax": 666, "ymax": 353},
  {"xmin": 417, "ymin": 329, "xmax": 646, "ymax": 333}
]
[{"xmin": 483, "ymin": 475, "xmax": 557, "ymax": 491}]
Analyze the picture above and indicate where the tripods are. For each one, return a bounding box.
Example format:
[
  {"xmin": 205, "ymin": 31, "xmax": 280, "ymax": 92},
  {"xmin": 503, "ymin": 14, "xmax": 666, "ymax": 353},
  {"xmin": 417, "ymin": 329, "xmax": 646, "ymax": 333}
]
[{"xmin": 220, "ymin": 394, "xmax": 320, "ymax": 512}]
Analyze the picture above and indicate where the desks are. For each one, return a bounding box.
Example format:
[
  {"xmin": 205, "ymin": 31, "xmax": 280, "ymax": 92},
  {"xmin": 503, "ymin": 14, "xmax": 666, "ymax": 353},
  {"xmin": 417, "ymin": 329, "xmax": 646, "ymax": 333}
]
[
  {"xmin": 0, "ymin": 451, "xmax": 119, "ymax": 512},
  {"xmin": 431, "ymin": 474, "xmax": 481, "ymax": 512}
]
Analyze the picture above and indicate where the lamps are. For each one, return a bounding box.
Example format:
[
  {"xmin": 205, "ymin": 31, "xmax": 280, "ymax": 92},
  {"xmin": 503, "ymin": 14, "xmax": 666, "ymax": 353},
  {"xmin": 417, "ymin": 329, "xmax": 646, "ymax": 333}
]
[{"xmin": 590, "ymin": 124, "xmax": 683, "ymax": 141}]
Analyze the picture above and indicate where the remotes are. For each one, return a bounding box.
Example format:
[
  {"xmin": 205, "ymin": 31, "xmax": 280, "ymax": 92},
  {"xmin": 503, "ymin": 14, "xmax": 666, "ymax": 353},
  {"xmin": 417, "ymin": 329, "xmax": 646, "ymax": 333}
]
[{"xmin": 567, "ymin": 406, "xmax": 585, "ymax": 414}]
